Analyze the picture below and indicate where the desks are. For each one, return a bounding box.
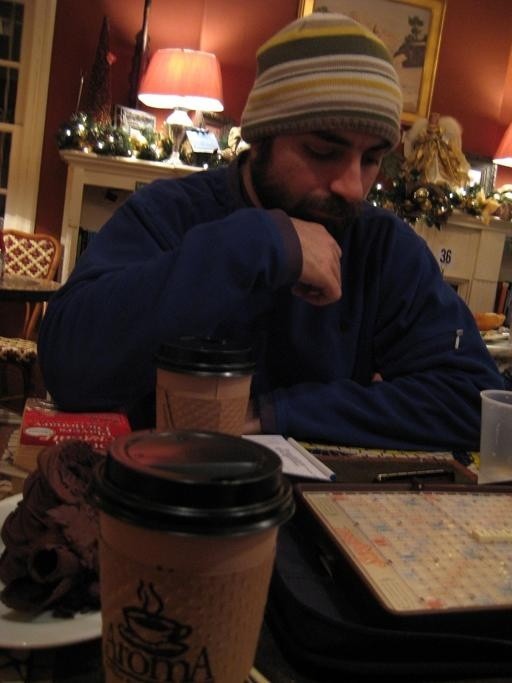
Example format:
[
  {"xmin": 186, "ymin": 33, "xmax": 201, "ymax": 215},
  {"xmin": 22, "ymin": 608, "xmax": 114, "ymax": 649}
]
[{"xmin": 56, "ymin": 150, "xmax": 509, "ymax": 320}]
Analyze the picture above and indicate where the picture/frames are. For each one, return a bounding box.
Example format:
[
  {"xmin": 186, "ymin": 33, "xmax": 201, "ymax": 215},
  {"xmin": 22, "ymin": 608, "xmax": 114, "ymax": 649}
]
[{"xmin": 298, "ymin": 0, "xmax": 447, "ymax": 126}]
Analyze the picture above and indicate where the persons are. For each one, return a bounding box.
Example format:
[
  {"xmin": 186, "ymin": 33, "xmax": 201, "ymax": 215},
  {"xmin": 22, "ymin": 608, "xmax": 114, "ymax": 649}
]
[
  {"xmin": 393, "ymin": 15, "xmax": 430, "ymax": 67},
  {"xmin": 36, "ymin": 10, "xmax": 509, "ymax": 454}
]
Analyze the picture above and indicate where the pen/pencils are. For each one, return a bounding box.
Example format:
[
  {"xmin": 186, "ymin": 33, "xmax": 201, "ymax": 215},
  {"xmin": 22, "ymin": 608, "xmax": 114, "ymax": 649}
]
[
  {"xmin": 287, "ymin": 436, "xmax": 337, "ymax": 481},
  {"xmin": 378, "ymin": 467, "xmax": 446, "ymax": 484}
]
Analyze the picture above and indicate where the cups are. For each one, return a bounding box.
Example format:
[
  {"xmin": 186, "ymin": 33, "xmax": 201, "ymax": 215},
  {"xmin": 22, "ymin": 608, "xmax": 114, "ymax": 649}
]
[
  {"xmin": 94, "ymin": 429, "xmax": 297, "ymax": 683},
  {"xmin": 151, "ymin": 334, "xmax": 262, "ymax": 431},
  {"xmin": 479, "ymin": 392, "xmax": 512, "ymax": 483}
]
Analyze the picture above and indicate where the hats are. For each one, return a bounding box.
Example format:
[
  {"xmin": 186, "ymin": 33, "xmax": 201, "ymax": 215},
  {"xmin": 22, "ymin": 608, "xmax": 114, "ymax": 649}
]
[{"xmin": 241, "ymin": 12, "xmax": 402, "ymax": 153}]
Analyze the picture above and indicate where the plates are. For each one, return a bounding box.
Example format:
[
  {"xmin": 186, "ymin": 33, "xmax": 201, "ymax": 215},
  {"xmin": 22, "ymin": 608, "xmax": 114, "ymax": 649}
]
[{"xmin": 1, "ymin": 493, "xmax": 102, "ymax": 652}]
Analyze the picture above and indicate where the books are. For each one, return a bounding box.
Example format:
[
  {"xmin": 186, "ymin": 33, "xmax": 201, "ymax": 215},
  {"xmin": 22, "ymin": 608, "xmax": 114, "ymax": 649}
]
[
  {"xmin": 16, "ymin": 397, "xmax": 133, "ymax": 473},
  {"xmin": 239, "ymin": 433, "xmax": 336, "ymax": 482}
]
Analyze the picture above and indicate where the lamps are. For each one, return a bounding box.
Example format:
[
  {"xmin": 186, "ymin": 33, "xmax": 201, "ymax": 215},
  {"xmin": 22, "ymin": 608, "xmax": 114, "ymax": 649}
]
[
  {"xmin": 493, "ymin": 122, "xmax": 512, "ymax": 168},
  {"xmin": 138, "ymin": 48, "xmax": 225, "ymax": 166}
]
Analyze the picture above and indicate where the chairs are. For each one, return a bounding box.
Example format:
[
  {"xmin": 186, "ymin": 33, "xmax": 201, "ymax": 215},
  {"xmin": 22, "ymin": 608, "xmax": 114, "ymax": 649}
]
[{"xmin": 1, "ymin": 230, "xmax": 63, "ymax": 400}]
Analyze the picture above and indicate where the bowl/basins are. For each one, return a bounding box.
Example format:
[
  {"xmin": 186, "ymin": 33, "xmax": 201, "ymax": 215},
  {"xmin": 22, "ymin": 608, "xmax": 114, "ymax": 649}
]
[{"xmin": 473, "ymin": 310, "xmax": 505, "ymax": 331}]
